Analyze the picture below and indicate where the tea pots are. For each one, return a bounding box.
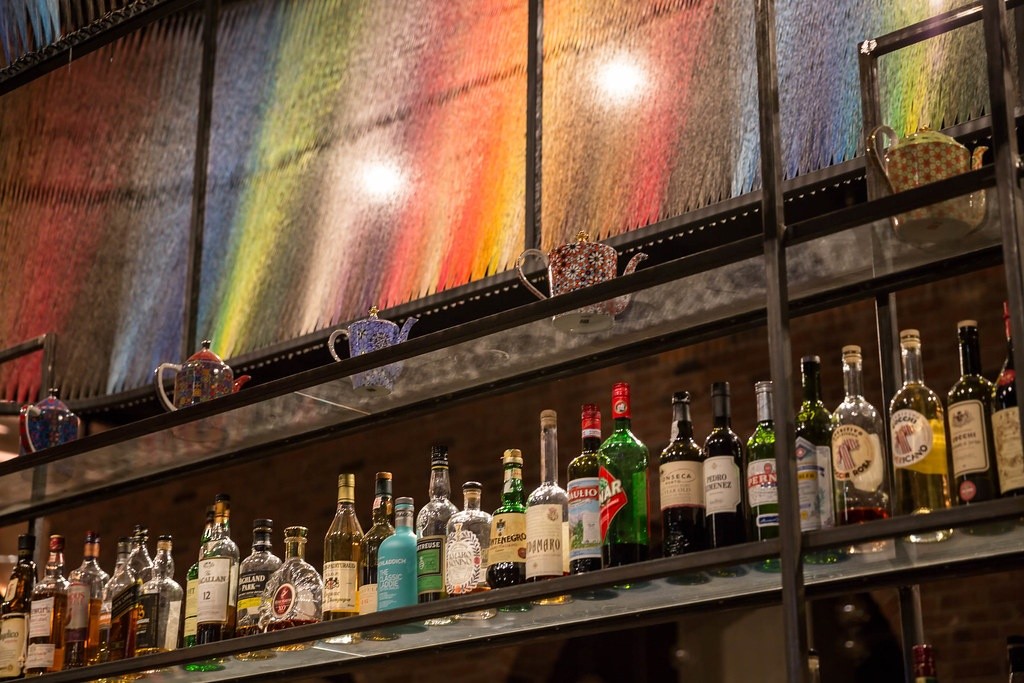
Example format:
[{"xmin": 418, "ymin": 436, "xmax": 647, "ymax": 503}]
[
  {"xmin": 865, "ymin": 123, "xmax": 988, "ymax": 245},
  {"xmin": 17, "ymin": 388, "xmax": 81, "ymax": 484},
  {"xmin": 516, "ymin": 231, "xmax": 650, "ymax": 336},
  {"xmin": 153, "ymin": 341, "xmax": 251, "ymax": 442},
  {"xmin": 328, "ymin": 305, "xmax": 419, "ymax": 397}
]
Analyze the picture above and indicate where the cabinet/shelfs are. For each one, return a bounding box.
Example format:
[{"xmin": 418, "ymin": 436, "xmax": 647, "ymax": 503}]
[{"xmin": 0, "ymin": 0, "xmax": 1024, "ymax": 683}]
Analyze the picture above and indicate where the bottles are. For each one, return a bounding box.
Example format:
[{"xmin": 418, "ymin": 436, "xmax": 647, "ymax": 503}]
[
  {"xmin": 745, "ymin": 381, "xmax": 783, "ymax": 575},
  {"xmin": 989, "ymin": 299, "xmax": 1024, "ymax": 526},
  {"xmin": 702, "ymin": 381, "xmax": 748, "ymax": 577},
  {"xmin": 946, "ymin": 319, "xmax": 1013, "ymax": 538},
  {"xmin": 787, "ymin": 356, "xmax": 850, "ymax": 566},
  {"xmin": 1, "ymin": 379, "xmax": 648, "ymax": 681},
  {"xmin": 659, "ymin": 391, "xmax": 709, "ymax": 585},
  {"xmin": 1006, "ymin": 635, "xmax": 1024, "ymax": 683},
  {"xmin": 886, "ymin": 327, "xmax": 953, "ymax": 547},
  {"xmin": 832, "ymin": 346, "xmax": 891, "ymax": 556},
  {"xmin": 807, "ymin": 649, "xmax": 820, "ymax": 683},
  {"xmin": 913, "ymin": 643, "xmax": 939, "ymax": 683}
]
